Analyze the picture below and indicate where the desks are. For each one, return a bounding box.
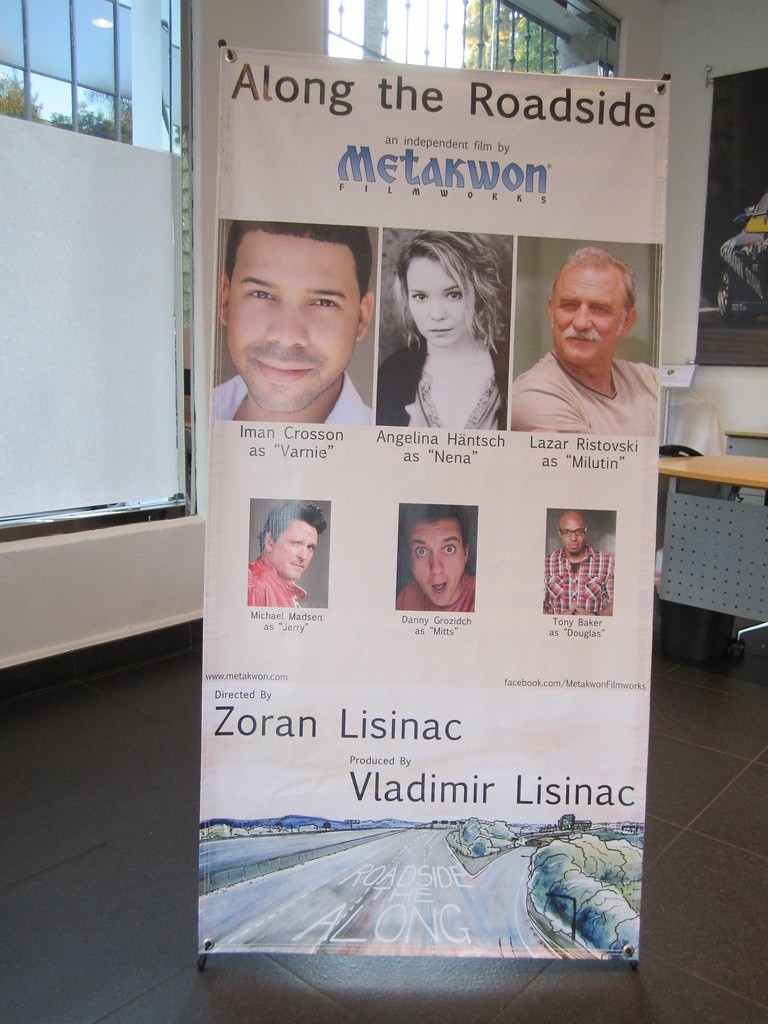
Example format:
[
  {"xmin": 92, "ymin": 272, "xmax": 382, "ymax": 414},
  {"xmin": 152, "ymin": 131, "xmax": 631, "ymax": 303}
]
[
  {"xmin": 723, "ymin": 431, "xmax": 768, "ymax": 460},
  {"xmin": 656, "ymin": 454, "xmax": 768, "ymax": 667}
]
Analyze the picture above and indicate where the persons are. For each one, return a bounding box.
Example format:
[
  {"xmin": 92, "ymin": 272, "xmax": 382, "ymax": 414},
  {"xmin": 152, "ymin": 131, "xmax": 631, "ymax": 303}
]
[
  {"xmin": 375, "ymin": 228, "xmax": 506, "ymax": 432},
  {"xmin": 545, "ymin": 510, "xmax": 614, "ymax": 616},
  {"xmin": 396, "ymin": 501, "xmax": 476, "ymax": 612},
  {"xmin": 212, "ymin": 220, "xmax": 376, "ymax": 425},
  {"xmin": 511, "ymin": 247, "xmax": 657, "ymax": 439},
  {"xmin": 247, "ymin": 501, "xmax": 327, "ymax": 608}
]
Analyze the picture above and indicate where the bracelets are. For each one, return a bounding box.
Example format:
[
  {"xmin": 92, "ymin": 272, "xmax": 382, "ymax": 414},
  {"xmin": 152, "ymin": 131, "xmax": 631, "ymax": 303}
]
[{"xmin": 592, "ymin": 612, "xmax": 599, "ymax": 616}]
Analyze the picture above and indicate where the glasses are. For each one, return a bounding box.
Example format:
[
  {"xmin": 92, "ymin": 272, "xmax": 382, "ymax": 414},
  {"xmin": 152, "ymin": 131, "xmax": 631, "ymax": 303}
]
[{"xmin": 559, "ymin": 525, "xmax": 587, "ymax": 536}]
